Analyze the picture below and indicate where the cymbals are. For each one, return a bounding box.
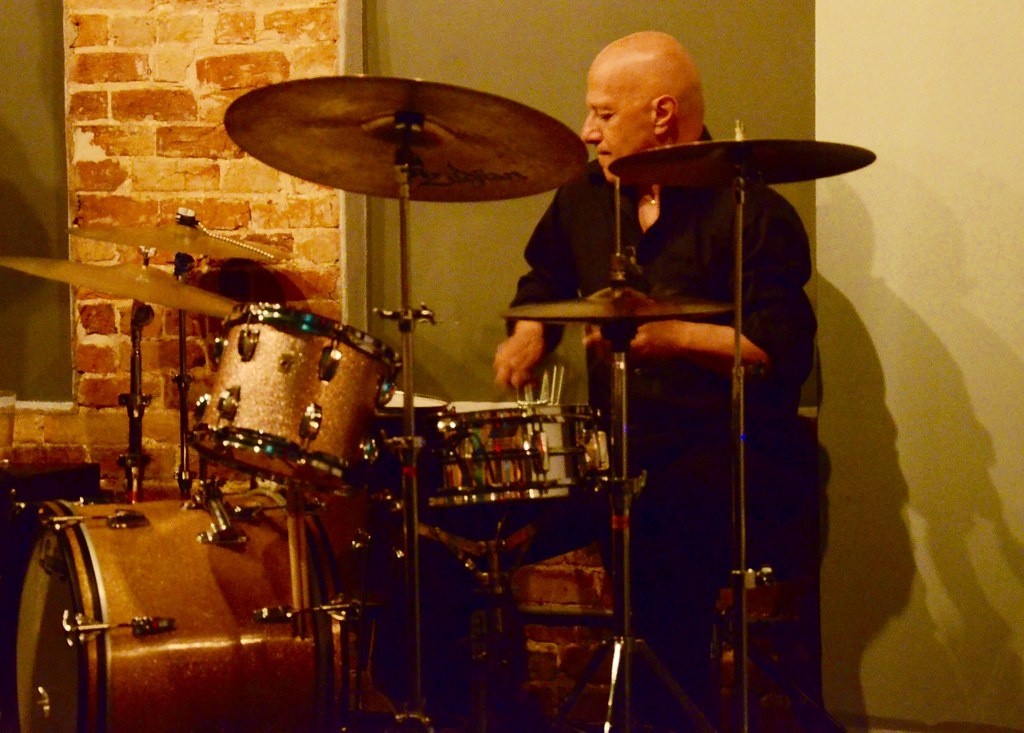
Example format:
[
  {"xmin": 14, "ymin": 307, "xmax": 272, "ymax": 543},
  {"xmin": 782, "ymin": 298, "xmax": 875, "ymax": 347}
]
[
  {"xmin": 221, "ymin": 73, "xmax": 592, "ymax": 206},
  {"xmin": 62, "ymin": 204, "xmax": 290, "ymax": 266},
  {"xmin": 499, "ymin": 252, "xmax": 737, "ymax": 323},
  {"xmin": 607, "ymin": 117, "xmax": 879, "ymax": 193},
  {"xmin": 1, "ymin": 246, "xmax": 244, "ymax": 321}
]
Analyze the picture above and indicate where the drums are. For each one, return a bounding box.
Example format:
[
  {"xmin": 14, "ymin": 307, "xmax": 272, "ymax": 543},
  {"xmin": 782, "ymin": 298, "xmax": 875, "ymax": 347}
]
[
  {"xmin": 408, "ymin": 402, "xmax": 604, "ymax": 508},
  {"xmin": 12, "ymin": 493, "xmax": 360, "ymax": 733},
  {"xmin": 185, "ymin": 299, "xmax": 405, "ymax": 497},
  {"xmin": 208, "ymin": 472, "xmax": 386, "ymax": 615}
]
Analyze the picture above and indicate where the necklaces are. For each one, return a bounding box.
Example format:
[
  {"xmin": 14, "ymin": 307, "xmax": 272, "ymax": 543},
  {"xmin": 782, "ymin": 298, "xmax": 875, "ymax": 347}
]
[{"xmin": 643, "ymin": 195, "xmax": 659, "ymax": 205}]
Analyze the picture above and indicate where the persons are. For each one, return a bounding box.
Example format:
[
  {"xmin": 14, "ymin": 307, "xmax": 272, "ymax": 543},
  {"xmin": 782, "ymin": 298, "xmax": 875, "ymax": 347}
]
[{"xmin": 491, "ymin": 31, "xmax": 831, "ymax": 733}]
